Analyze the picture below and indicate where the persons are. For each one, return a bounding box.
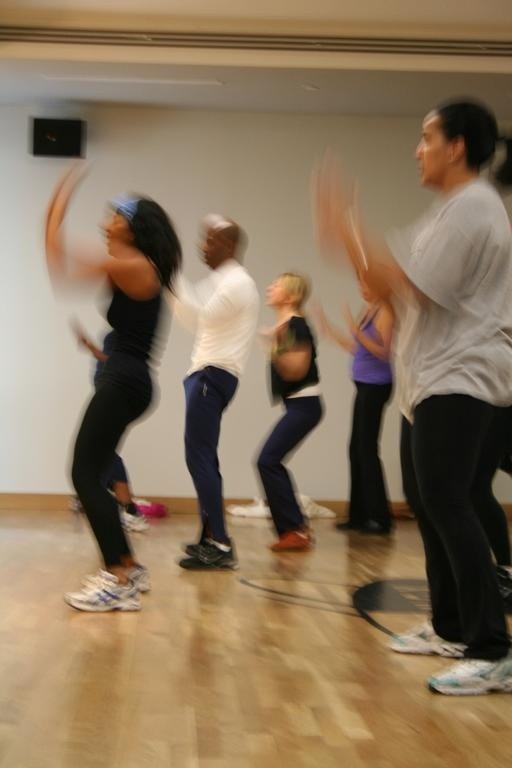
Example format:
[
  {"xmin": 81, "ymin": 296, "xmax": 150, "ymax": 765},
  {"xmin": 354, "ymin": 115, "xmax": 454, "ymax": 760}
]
[
  {"xmin": 45, "ymin": 159, "xmax": 182, "ymax": 611},
  {"xmin": 164, "ymin": 217, "xmax": 259, "ymax": 572},
  {"xmin": 73, "ymin": 325, "xmax": 149, "ymax": 533},
  {"xmin": 472, "ymin": 408, "xmax": 512, "ymax": 598},
  {"xmin": 316, "ymin": 274, "xmax": 395, "ymax": 533},
  {"xmin": 251, "ymin": 273, "xmax": 323, "ymax": 551},
  {"xmin": 309, "ymin": 99, "xmax": 512, "ymax": 696}
]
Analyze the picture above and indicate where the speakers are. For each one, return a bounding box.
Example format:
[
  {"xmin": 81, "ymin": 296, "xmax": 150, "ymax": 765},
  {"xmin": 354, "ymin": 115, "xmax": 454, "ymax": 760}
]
[{"xmin": 33, "ymin": 119, "xmax": 81, "ymax": 156}]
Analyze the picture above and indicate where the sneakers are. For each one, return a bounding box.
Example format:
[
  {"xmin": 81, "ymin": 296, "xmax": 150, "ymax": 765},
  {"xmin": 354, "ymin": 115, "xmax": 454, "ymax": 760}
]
[
  {"xmin": 270, "ymin": 522, "xmax": 317, "ymax": 554},
  {"xmin": 105, "ymin": 508, "xmax": 151, "ymax": 533},
  {"xmin": 178, "ymin": 535, "xmax": 239, "ymax": 573},
  {"xmin": 426, "ymin": 651, "xmax": 512, "ymax": 699},
  {"xmin": 335, "ymin": 507, "xmax": 395, "ymax": 538},
  {"xmin": 60, "ymin": 567, "xmax": 144, "ymax": 615},
  {"xmin": 387, "ymin": 616, "xmax": 469, "ymax": 659}
]
